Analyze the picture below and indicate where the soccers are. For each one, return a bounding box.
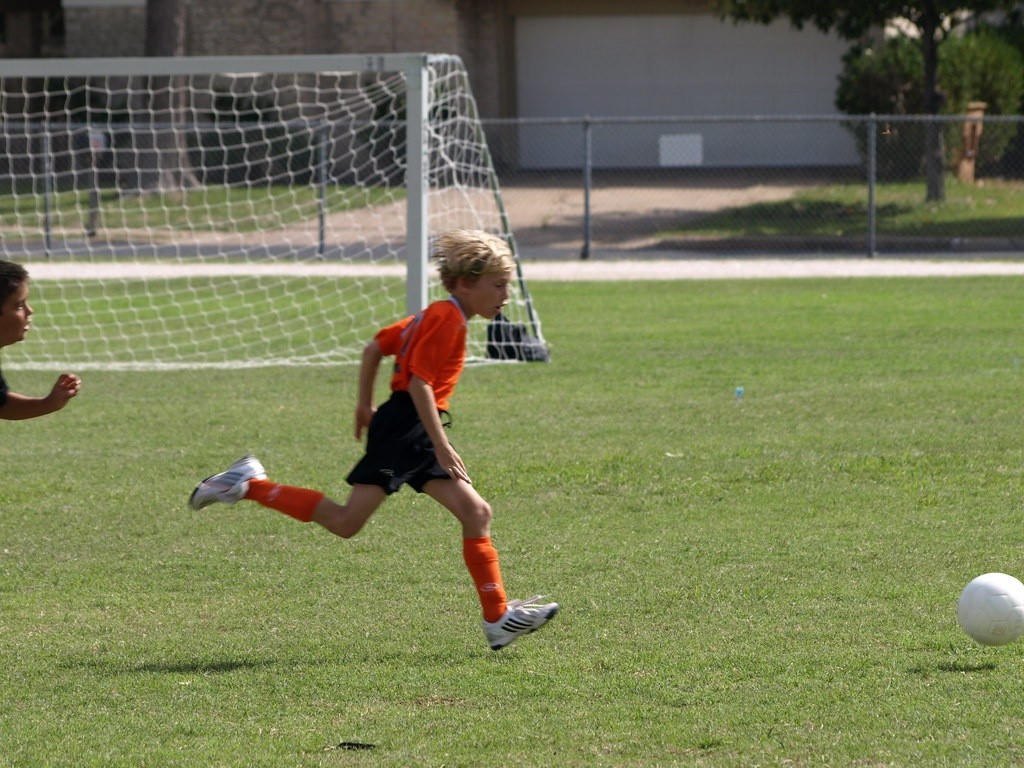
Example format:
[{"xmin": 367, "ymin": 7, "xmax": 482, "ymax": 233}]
[{"xmin": 954, "ymin": 572, "xmax": 1024, "ymax": 646}]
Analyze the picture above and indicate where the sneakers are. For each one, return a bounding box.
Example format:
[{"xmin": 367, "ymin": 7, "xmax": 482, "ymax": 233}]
[
  {"xmin": 482, "ymin": 593, "xmax": 559, "ymax": 650},
  {"xmin": 189, "ymin": 456, "xmax": 267, "ymax": 511}
]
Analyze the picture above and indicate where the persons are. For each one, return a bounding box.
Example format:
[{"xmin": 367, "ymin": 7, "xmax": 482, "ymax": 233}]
[
  {"xmin": 0, "ymin": 259, "xmax": 80, "ymax": 420},
  {"xmin": 187, "ymin": 229, "xmax": 559, "ymax": 652}
]
